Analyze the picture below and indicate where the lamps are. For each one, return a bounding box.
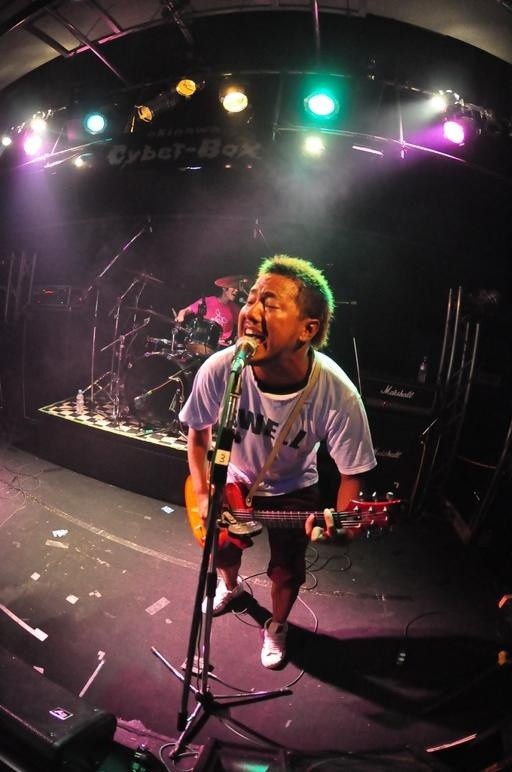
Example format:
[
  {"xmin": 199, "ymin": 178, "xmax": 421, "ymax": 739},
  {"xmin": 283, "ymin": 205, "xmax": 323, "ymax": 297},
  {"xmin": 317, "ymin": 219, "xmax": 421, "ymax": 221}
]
[
  {"xmin": 304, "ymin": 81, "xmax": 338, "ymax": 117},
  {"xmin": 136, "ymin": 87, "xmax": 172, "ymax": 122},
  {"xmin": 444, "ymin": 105, "xmax": 486, "ymax": 146},
  {"xmin": 178, "ymin": 76, "xmax": 204, "ymax": 99},
  {"xmin": 220, "ymin": 76, "xmax": 248, "ymax": 113},
  {"xmin": 82, "ymin": 114, "xmax": 116, "ymax": 135}
]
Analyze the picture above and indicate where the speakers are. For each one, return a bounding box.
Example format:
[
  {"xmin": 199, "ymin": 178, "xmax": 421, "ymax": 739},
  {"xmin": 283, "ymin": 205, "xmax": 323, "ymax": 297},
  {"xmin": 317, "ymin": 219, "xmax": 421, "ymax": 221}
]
[
  {"xmin": 351, "ymin": 378, "xmax": 441, "ymax": 507},
  {"xmin": 0, "ymin": 645, "xmax": 117, "ymax": 772}
]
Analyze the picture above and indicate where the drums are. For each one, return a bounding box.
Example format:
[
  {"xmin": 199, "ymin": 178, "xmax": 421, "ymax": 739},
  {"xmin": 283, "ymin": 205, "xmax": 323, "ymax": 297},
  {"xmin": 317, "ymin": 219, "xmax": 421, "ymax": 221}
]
[
  {"xmin": 184, "ymin": 317, "xmax": 223, "ymax": 357},
  {"xmin": 123, "ymin": 352, "xmax": 197, "ymax": 430},
  {"xmin": 143, "ymin": 336, "xmax": 185, "ymax": 355}
]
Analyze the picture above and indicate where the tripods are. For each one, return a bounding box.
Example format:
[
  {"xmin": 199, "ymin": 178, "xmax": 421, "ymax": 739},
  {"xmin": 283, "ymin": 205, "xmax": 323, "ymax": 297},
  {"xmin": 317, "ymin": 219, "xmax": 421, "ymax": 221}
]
[
  {"xmin": 71, "ymin": 230, "xmax": 188, "ymax": 443},
  {"xmin": 151, "ymin": 372, "xmax": 291, "ymax": 760}
]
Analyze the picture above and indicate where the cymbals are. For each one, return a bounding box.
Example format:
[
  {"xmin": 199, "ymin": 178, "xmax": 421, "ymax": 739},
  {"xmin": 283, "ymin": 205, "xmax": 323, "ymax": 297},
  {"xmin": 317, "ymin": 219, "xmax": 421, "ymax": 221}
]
[
  {"xmin": 123, "ymin": 306, "xmax": 174, "ymax": 325},
  {"xmin": 214, "ymin": 274, "xmax": 255, "ymax": 290},
  {"xmin": 123, "ymin": 267, "xmax": 165, "ymax": 286}
]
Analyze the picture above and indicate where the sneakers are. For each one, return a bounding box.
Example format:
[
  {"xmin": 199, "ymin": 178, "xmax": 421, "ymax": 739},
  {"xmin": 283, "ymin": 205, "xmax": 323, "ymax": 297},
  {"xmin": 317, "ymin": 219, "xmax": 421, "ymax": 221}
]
[
  {"xmin": 260, "ymin": 617, "xmax": 289, "ymax": 669},
  {"xmin": 201, "ymin": 574, "xmax": 244, "ymax": 616}
]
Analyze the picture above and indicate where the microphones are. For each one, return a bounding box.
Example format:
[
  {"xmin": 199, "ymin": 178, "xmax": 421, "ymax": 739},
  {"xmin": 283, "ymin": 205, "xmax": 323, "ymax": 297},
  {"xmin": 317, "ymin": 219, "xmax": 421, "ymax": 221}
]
[
  {"xmin": 228, "ymin": 336, "xmax": 259, "ymax": 375},
  {"xmin": 253, "ymin": 215, "xmax": 259, "ymax": 239}
]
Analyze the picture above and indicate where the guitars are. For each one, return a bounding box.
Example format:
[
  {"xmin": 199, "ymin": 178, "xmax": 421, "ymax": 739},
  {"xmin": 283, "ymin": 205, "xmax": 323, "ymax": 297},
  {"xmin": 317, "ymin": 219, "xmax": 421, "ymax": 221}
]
[{"xmin": 185, "ymin": 473, "xmax": 410, "ymax": 553}]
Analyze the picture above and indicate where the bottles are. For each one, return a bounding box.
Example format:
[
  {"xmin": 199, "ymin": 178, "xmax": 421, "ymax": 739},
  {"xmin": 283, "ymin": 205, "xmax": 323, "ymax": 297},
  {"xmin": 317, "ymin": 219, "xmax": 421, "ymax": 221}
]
[
  {"xmin": 76, "ymin": 389, "xmax": 85, "ymax": 415},
  {"xmin": 417, "ymin": 356, "xmax": 429, "ymax": 383}
]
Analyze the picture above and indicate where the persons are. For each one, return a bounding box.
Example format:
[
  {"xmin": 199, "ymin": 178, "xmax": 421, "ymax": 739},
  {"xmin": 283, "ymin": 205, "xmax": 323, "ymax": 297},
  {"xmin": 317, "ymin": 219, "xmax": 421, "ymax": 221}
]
[
  {"xmin": 177, "ymin": 250, "xmax": 379, "ymax": 671},
  {"xmin": 174, "ymin": 285, "xmax": 242, "ymax": 394}
]
